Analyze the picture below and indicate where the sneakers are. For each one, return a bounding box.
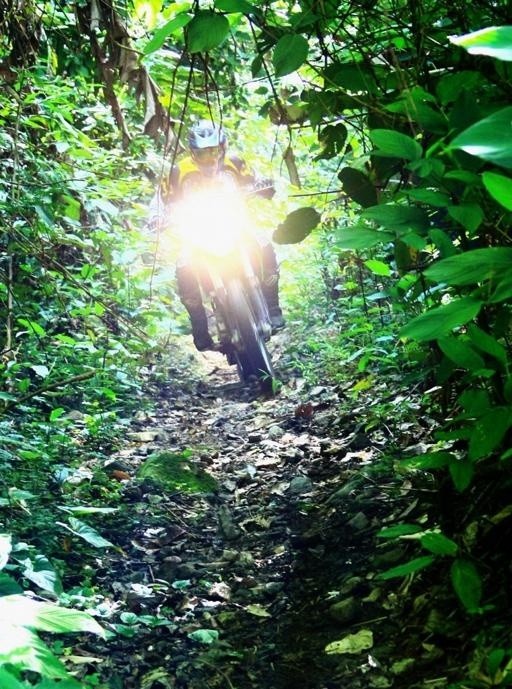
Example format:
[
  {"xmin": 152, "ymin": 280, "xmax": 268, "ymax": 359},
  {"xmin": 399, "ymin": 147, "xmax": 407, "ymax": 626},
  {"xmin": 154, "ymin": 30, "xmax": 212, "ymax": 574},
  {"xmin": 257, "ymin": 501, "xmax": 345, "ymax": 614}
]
[
  {"xmin": 192, "ymin": 327, "xmax": 213, "ymax": 352},
  {"xmin": 268, "ymin": 304, "xmax": 286, "ymax": 332}
]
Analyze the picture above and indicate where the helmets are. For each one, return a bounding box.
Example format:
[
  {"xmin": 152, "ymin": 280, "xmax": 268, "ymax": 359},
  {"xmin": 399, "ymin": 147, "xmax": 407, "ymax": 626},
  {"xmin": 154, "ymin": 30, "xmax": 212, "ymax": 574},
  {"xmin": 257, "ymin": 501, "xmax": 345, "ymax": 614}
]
[{"xmin": 186, "ymin": 118, "xmax": 230, "ymax": 173}]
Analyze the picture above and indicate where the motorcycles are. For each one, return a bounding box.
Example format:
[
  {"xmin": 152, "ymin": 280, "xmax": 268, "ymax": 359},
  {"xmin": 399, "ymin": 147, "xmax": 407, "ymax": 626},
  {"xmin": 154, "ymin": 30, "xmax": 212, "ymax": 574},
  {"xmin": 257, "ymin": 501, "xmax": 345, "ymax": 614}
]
[{"xmin": 147, "ymin": 156, "xmax": 291, "ymax": 393}]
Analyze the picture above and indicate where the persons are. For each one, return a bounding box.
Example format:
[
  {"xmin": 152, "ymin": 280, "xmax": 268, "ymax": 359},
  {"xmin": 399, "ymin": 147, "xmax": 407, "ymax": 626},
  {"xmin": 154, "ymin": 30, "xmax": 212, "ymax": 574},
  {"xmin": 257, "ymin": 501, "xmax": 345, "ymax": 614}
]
[{"xmin": 155, "ymin": 116, "xmax": 288, "ymax": 351}]
